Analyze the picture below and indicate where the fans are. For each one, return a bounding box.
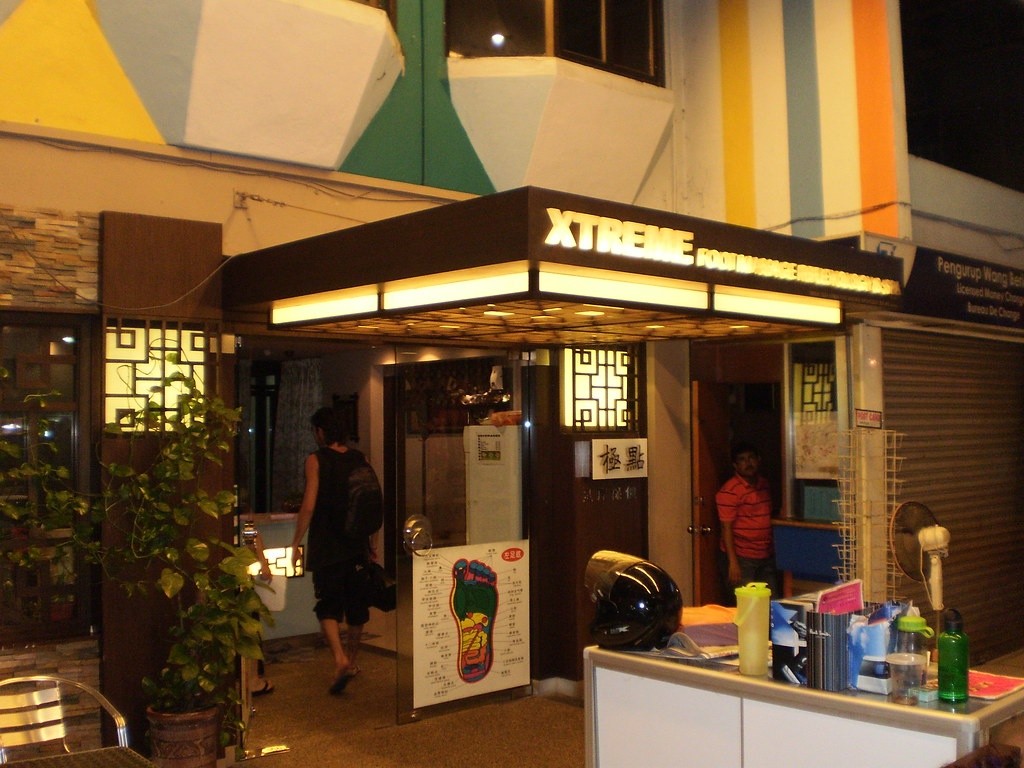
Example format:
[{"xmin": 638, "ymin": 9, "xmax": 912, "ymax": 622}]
[{"xmin": 887, "ymin": 499, "xmax": 950, "ymax": 662}]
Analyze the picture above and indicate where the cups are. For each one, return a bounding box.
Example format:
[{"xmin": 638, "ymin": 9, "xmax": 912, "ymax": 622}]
[
  {"xmin": 897, "ymin": 616, "xmax": 934, "ymax": 656},
  {"xmin": 733, "ymin": 582, "xmax": 771, "ymax": 676},
  {"xmin": 886, "ymin": 653, "xmax": 927, "ymax": 706}
]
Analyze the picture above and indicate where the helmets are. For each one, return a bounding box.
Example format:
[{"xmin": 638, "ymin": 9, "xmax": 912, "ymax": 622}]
[{"xmin": 583, "ymin": 550, "xmax": 682, "ymax": 649}]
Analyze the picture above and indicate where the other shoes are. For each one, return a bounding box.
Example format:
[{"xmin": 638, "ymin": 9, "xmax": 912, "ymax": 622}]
[{"xmin": 328, "ymin": 665, "xmax": 351, "ymax": 694}]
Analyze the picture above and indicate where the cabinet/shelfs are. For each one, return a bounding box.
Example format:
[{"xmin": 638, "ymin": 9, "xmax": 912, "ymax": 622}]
[{"xmin": 583, "ymin": 646, "xmax": 1023, "ymax": 768}]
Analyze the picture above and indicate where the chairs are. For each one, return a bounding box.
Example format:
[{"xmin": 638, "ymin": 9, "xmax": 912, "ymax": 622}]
[{"xmin": 0, "ymin": 676, "xmax": 159, "ymax": 768}]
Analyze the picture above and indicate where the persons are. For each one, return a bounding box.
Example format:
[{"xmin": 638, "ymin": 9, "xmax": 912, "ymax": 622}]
[
  {"xmin": 238, "ymin": 469, "xmax": 277, "ymax": 695},
  {"xmin": 291, "ymin": 406, "xmax": 385, "ymax": 696},
  {"xmin": 716, "ymin": 433, "xmax": 777, "ymax": 604}
]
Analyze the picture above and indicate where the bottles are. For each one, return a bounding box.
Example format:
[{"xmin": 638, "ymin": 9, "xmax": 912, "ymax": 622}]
[{"xmin": 938, "ymin": 610, "xmax": 969, "ymax": 704}]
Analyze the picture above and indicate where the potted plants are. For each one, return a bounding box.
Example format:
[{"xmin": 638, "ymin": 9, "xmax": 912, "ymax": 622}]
[{"xmin": 0, "ymin": 340, "xmax": 277, "ymax": 768}]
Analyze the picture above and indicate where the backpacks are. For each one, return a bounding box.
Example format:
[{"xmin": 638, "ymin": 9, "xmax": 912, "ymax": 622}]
[{"xmin": 321, "ymin": 448, "xmax": 384, "ymax": 540}]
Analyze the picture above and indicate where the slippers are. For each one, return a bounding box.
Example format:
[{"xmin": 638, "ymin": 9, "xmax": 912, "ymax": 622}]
[{"xmin": 250, "ymin": 679, "xmax": 274, "ymax": 696}]
